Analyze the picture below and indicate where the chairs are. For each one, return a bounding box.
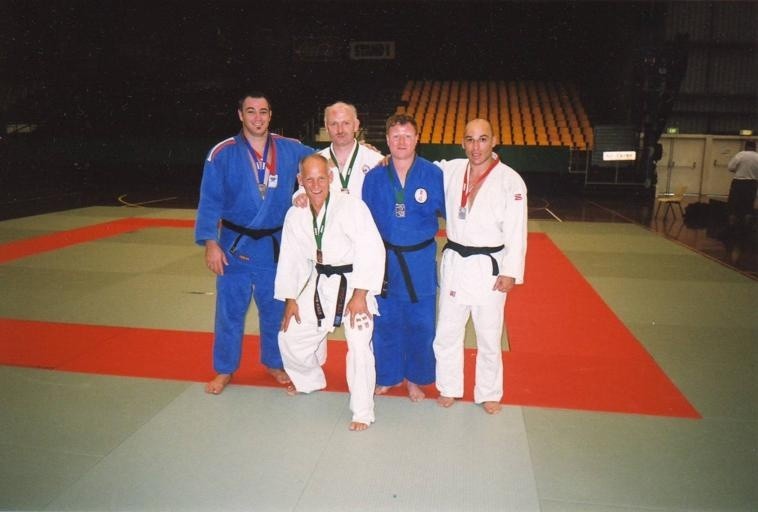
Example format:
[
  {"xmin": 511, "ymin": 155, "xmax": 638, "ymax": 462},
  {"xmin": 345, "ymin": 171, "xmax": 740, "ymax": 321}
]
[{"xmin": 395, "ymin": 78, "xmax": 594, "ymax": 152}]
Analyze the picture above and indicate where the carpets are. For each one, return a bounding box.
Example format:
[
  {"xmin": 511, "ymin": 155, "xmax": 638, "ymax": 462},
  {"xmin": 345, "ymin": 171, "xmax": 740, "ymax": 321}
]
[{"xmin": 0, "ymin": 217, "xmax": 702, "ymax": 421}]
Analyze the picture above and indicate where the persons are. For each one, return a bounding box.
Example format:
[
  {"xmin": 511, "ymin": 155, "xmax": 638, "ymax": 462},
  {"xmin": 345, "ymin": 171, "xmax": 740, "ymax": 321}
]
[
  {"xmin": 273, "ymin": 154, "xmax": 387, "ymax": 431},
  {"xmin": 378, "ymin": 119, "xmax": 528, "ymax": 415},
  {"xmin": 292, "ymin": 101, "xmax": 385, "ymax": 367},
  {"xmin": 362, "ymin": 114, "xmax": 446, "ymax": 402},
  {"xmin": 717, "ymin": 141, "xmax": 758, "ymax": 248},
  {"xmin": 195, "ymin": 91, "xmax": 381, "ymax": 395}
]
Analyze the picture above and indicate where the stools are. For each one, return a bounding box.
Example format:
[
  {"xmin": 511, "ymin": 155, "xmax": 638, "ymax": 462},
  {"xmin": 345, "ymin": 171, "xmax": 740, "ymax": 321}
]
[{"xmin": 656, "ymin": 197, "xmax": 683, "ymax": 219}]
